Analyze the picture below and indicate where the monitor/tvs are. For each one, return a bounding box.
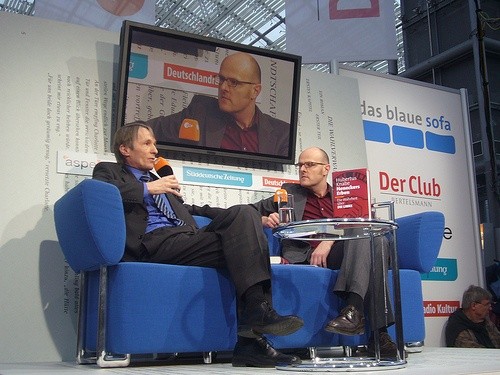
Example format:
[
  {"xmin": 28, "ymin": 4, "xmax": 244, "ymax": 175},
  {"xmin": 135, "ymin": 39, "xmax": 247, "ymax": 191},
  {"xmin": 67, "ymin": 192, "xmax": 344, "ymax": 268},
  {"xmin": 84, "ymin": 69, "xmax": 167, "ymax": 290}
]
[{"xmin": 113, "ymin": 20, "xmax": 302, "ymax": 164}]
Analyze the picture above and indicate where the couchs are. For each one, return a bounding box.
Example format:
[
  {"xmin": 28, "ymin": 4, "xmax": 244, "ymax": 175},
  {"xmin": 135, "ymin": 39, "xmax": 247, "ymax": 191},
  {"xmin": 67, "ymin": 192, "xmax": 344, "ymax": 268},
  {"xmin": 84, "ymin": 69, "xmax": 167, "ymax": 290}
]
[
  {"xmin": 260, "ymin": 211, "xmax": 445, "ymax": 353},
  {"xmin": 53, "ymin": 178, "xmax": 237, "ymax": 370}
]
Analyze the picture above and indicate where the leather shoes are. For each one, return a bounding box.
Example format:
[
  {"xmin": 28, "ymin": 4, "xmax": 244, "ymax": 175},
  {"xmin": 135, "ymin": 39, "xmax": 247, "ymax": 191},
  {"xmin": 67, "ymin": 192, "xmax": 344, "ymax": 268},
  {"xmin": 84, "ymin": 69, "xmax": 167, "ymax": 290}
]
[
  {"xmin": 231, "ymin": 337, "xmax": 302, "ymax": 367},
  {"xmin": 323, "ymin": 304, "xmax": 367, "ymax": 336},
  {"xmin": 366, "ymin": 332, "xmax": 397, "ymax": 358},
  {"xmin": 237, "ymin": 296, "xmax": 306, "ymax": 337}
]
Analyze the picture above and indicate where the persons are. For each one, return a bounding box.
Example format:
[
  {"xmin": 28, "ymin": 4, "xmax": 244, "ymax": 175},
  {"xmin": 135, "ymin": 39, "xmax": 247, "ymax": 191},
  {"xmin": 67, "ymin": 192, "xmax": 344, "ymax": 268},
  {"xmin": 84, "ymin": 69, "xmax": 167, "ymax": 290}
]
[
  {"xmin": 252, "ymin": 146, "xmax": 409, "ymax": 357},
  {"xmin": 147, "ymin": 52, "xmax": 290, "ymax": 157},
  {"xmin": 92, "ymin": 122, "xmax": 303, "ymax": 368},
  {"xmin": 445, "ymin": 287, "xmax": 500, "ymax": 348}
]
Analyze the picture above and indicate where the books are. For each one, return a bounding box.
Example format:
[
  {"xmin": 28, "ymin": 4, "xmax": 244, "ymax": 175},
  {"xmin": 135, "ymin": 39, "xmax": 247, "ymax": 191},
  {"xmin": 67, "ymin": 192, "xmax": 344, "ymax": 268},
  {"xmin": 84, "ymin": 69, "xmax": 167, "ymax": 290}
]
[
  {"xmin": 269, "ymin": 256, "xmax": 289, "ymax": 264},
  {"xmin": 332, "ymin": 168, "xmax": 371, "ymax": 219}
]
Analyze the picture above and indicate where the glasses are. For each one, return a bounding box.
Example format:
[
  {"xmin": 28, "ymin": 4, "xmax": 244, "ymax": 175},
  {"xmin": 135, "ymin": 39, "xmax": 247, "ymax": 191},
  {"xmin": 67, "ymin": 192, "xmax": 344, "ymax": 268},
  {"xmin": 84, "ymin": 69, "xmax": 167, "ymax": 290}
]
[
  {"xmin": 295, "ymin": 161, "xmax": 327, "ymax": 169},
  {"xmin": 214, "ymin": 75, "xmax": 258, "ymax": 87},
  {"xmin": 474, "ymin": 300, "xmax": 493, "ymax": 308}
]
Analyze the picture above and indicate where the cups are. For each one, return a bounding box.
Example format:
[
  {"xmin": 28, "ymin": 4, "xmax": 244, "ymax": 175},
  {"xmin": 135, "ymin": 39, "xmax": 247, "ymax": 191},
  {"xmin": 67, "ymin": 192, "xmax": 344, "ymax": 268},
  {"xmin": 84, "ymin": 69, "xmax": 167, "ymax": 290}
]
[{"xmin": 278, "ymin": 194, "xmax": 295, "ymax": 226}]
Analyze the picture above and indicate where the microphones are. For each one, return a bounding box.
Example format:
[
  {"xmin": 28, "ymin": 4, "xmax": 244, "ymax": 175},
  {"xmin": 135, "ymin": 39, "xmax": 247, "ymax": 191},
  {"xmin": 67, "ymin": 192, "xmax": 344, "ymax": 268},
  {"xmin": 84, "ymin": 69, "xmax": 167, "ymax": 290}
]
[
  {"xmin": 178, "ymin": 119, "xmax": 201, "ymax": 145},
  {"xmin": 152, "ymin": 158, "xmax": 185, "ymax": 203},
  {"xmin": 273, "ymin": 189, "xmax": 287, "ymax": 214}
]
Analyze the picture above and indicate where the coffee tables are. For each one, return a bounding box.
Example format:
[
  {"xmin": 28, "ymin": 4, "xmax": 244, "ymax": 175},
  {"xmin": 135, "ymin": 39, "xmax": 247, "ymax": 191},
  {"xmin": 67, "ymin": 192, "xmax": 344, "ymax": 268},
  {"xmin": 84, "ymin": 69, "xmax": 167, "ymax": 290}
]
[{"xmin": 273, "ymin": 219, "xmax": 409, "ymax": 372}]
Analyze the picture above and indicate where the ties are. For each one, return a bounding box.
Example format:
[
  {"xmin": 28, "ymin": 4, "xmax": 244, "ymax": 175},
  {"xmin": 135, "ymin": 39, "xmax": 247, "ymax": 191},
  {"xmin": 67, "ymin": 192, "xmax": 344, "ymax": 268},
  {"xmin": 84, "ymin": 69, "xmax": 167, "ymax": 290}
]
[{"xmin": 139, "ymin": 176, "xmax": 186, "ymax": 227}]
